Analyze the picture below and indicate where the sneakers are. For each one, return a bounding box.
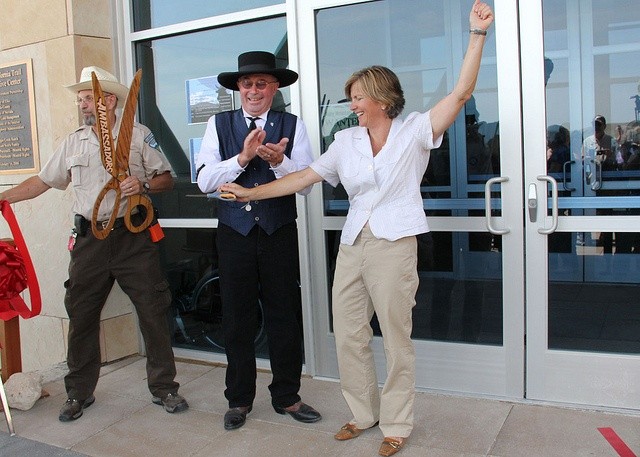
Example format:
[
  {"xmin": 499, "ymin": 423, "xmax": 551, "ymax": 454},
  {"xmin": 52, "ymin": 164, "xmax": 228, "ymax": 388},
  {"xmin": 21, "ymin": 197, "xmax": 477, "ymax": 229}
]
[
  {"xmin": 59, "ymin": 394, "xmax": 95, "ymax": 422},
  {"xmin": 152, "ymin": 392, "xmax": 188, "ymax": 413}
]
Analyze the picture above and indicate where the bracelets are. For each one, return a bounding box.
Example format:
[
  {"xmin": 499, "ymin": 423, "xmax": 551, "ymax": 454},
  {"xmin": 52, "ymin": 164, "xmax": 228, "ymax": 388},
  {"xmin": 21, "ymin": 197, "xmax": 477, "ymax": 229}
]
[{"xmin": 469, "ymin": 28, "xmax": 488, "ymax": 36}]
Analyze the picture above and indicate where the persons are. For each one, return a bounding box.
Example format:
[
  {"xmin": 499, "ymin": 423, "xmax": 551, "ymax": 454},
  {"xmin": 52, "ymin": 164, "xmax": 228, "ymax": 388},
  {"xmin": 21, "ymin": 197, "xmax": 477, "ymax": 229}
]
[
  {"xmin": 1, "ymin": 66, "xmax": 189, "ymax": 422},
  {"xmin": 196, "ymin": 50, "xmax": 321, "ymax": 430},
  {"xmin": 464, "ymin": 57, "xmax": 554, "ymax": 174},
  {"xmin": 220, "ymin": 1, "xmax": 495, "ymax": 457},
  {"xmin": 616, "ymin": 120, "xmax": 640, "ymax": 253},
  {"xmin": 570, "ymin": 130, "xmax": 592, "ymax": 246},
  {"xmin": 581, "ymin": 116, "xmax": 624, "ymax": 172},
  {"xmin": 547, "ymin": 125, "xmax": 572, "ymax": 252}
]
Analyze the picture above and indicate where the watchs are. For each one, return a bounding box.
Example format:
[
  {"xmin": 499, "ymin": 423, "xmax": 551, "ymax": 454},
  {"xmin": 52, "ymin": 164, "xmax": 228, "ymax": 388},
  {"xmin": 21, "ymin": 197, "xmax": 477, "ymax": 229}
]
[{"xmin": 142, "ymin": 182, "xmax": 151, "ymax": 193}]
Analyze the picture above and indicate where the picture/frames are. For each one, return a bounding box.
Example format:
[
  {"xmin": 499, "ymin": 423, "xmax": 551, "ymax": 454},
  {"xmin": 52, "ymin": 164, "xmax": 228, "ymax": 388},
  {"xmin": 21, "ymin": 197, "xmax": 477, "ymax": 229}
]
[{"xmin": 0, "ymin": 59, "xmax": 41, "ymax": 174}]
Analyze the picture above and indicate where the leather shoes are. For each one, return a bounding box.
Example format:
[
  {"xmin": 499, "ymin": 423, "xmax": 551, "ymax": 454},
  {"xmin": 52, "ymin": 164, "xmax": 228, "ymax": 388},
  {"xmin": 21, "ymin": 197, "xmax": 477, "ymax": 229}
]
[
  {"xmin": 224, "ymin": 405, "xmax": 253, "ymax": 430},
  {"xmin": 275, "ymin": 400, "xmax": 322, "ymax": 423},
  {"xmin": 334, "ymin": 420, "xmax": 379, "ymax": 441},
  {"xmin": 378, "ymin": 437, "xmax": 405, "ymax": 456}
]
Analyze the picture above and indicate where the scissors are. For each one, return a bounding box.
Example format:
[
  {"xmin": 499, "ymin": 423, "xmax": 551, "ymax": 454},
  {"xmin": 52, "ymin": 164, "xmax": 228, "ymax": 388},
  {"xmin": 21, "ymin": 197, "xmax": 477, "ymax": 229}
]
[{"xmin": 91, "ymin": 69, "xmax": 154, "ymax": 240}]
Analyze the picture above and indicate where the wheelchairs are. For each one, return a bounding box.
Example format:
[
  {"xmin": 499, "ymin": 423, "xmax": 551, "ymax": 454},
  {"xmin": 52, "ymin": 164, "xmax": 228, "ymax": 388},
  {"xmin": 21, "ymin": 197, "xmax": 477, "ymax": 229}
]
[{"xmin": 162, "ymin": 227, "xmax": 266, "ymax": 353}]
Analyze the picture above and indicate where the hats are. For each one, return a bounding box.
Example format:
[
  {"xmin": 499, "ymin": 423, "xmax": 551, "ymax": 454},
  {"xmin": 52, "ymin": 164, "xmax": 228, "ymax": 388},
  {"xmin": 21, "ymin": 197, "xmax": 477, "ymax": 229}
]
[
  {"xmin": 63, "ymin": 66, "xmax": 130, "ymax": 109},
  {"xmin": 217, "ymin": 51, "xmax": 298, "ymax": 91}
]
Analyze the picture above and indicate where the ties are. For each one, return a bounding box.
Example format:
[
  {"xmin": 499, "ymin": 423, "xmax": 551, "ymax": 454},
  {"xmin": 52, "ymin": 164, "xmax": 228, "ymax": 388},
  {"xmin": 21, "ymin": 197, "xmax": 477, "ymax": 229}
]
[{"xmin": 246, "ymin": 117, "xmax": 262, "ymax": 171}]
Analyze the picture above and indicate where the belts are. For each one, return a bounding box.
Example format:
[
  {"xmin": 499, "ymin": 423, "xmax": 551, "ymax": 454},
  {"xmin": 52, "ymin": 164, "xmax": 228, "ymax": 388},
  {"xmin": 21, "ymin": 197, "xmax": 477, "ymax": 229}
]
[{"xmin": 87, "ymin": 212, "xmax": 142, "ymax": 231}]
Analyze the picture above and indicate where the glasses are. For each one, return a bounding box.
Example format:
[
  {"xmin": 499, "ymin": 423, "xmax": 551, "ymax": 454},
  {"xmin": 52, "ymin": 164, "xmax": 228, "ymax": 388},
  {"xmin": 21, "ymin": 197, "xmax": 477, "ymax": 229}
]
[
  {"xmin": 242, "ymin": 79, "xmax": 277, "ymax": 90},
  {"xmin": 74, "ymin": 94, "xmax": 111, "ymax": 105}
]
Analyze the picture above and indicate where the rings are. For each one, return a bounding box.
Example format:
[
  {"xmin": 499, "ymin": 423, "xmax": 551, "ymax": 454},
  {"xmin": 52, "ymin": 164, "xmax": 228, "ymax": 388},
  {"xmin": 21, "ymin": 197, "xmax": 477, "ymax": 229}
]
[
  {"xmin": 129, "ymin": 188, "xmax": 134, "ymax": 192},
  {"xmin": 268, "ymin": 155, "xmax": 272, "ymax": 158}
]
